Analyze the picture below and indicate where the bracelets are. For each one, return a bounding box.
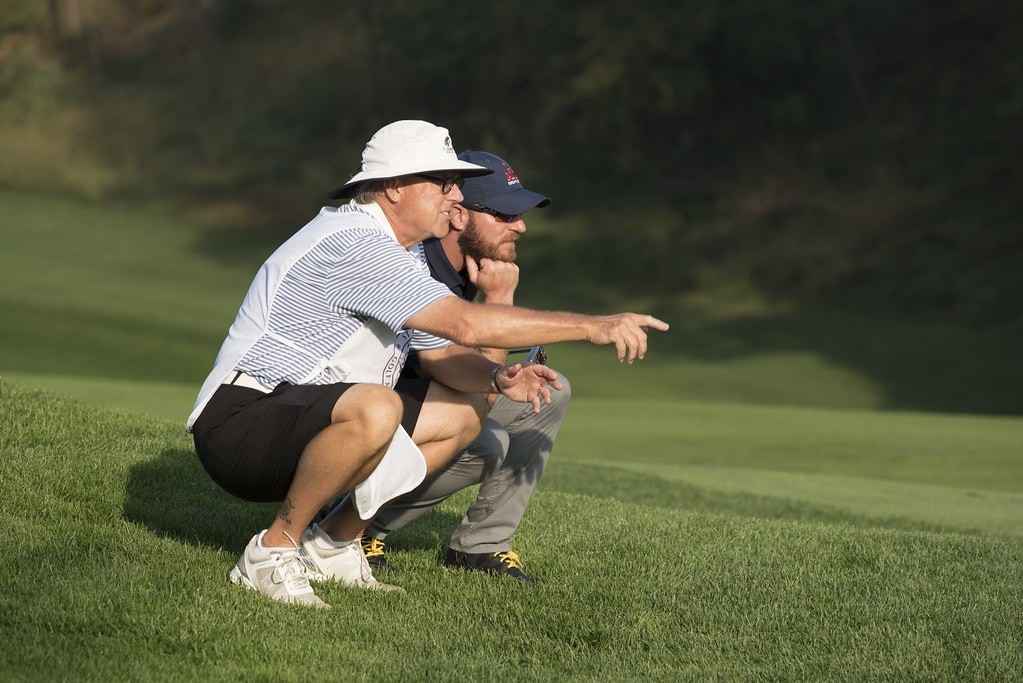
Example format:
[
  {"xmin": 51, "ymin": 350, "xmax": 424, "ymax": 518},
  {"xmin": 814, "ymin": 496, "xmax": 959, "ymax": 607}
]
[{"xmin": 490, "ymin": 366, "xmax": 502, "ymax": 394}]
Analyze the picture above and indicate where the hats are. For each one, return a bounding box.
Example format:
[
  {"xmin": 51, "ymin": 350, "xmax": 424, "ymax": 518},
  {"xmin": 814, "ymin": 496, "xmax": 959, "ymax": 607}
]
[
  {"xmin": 453, "ymin": 149, "xmax": 552, "ymax": 215},
  {"xmin": 327, "ymin": 119, "xmax": 493, "ymax": 200}
]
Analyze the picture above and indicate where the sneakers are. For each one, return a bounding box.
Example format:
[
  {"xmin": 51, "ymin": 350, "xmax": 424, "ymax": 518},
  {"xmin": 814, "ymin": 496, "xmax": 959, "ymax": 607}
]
[
  {"xmin": 225, "ymin": 528, "xmax": 331, "ymax": 610},
  {"xmin": 300, "ymin": 531, "xmax": 406, "ymax": 591},
  {"xmin": 445, "ymin": 549, "xmax": 527, "ymax": 580},
  {"xmin": 360, "ymin": 534, "xmax": 396, "ymax": 572}
]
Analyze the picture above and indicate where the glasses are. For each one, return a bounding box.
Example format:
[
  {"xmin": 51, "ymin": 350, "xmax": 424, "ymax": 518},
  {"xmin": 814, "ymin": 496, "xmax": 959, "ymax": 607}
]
[
  {"xmin": 480, "ymin": 207, "xmax": 521, "ymax": 222},
  {"xmin": 417, "ymin": 173, "xmax": 466, "ymax": 194}
]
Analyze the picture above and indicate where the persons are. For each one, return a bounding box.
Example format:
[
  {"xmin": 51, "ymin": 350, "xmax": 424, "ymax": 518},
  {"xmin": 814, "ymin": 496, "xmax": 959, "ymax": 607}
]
[
  {"xmin": 361, "ymin": 150, "xmax": 572, "ymax": 585},
  {"xmin": 186, "ymin": 120, "xmax": 670, "ymax": 609}
]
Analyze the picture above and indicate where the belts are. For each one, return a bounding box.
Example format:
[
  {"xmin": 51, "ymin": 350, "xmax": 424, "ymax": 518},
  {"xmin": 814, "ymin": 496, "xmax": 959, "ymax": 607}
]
[{"xmin": 221, "ymin": 371, "xmax": 274, "ymax": 394}]
